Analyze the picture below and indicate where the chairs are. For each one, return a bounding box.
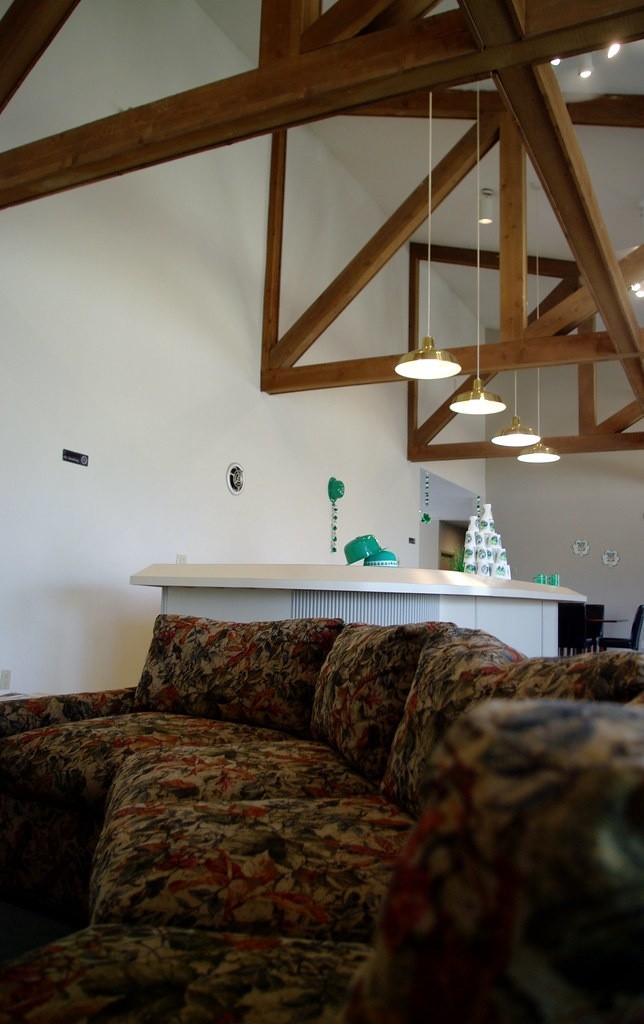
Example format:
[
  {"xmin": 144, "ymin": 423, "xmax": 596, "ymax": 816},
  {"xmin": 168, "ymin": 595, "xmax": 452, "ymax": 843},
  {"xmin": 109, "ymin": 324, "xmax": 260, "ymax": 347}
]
[{"xmin": 558, "ymin": 602, "xmax": 644, "ymax": 656}]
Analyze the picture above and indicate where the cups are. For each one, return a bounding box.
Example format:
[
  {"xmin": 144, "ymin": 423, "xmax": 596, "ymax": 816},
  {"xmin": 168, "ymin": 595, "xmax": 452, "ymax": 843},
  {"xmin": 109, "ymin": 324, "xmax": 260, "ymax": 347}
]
[
  {"xmin": 463, "ymin": 504, "xmax": 511, "ymax": 579},
  {"xmin": 532, "ymin": 573, "xmax": 547, "ymax": 584},
  {"xmin": 548, "ymin": 574, "xmax": 560, "ymax": 586}
]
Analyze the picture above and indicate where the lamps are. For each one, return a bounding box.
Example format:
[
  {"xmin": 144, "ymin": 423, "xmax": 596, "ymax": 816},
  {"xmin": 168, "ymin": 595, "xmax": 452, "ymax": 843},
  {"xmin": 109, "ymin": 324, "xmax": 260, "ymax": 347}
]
[
  {"xmin": 518, "ymin": 180, "xmax": 561, "ymax": 464},
  {"xmin": 492, "ymin": 368, "xmax": 541, "ymax": 446},
  {"xmin": 450, "ymin": 77, "xmax": 508, "ymax": 416},
  {"xmin": 578, "ymin": 54, "xmax": 592, "ymax": 78},
  {"xmin": 394, "ymin": 92, "xmax": 463, "ymax": 380}
]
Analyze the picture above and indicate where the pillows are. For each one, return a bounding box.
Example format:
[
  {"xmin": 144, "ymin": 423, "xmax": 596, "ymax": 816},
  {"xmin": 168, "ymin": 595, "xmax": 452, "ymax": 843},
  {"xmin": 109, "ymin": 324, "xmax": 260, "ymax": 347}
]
[
  {"xmin": 331, "ymin": 697, "xmax": 644, "ymax": 1022},
  {"xmin": 309, "ymin": 622, "xmax": 456, "ymax": 783},
  {"xmin": 379, "ymin": 622, "xmax": 643, "ymax": 818},
  {"xmin": 134, "ymin": 617, "xmax": 347, "ymax": 736}
]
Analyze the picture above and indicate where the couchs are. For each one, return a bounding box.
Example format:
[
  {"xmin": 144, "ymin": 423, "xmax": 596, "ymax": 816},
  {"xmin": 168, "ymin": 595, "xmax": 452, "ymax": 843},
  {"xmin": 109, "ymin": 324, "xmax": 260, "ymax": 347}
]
[{"xmin": 0, "ymin": 615, "xmax": 644, "ymax": 1024}]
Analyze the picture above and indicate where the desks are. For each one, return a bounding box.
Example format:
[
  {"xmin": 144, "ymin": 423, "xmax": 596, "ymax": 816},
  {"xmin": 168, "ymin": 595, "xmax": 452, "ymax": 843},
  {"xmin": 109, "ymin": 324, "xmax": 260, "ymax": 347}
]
[{"xmin": 584, "ymin": 619, "xmax": 627, "ymax": 653}]
